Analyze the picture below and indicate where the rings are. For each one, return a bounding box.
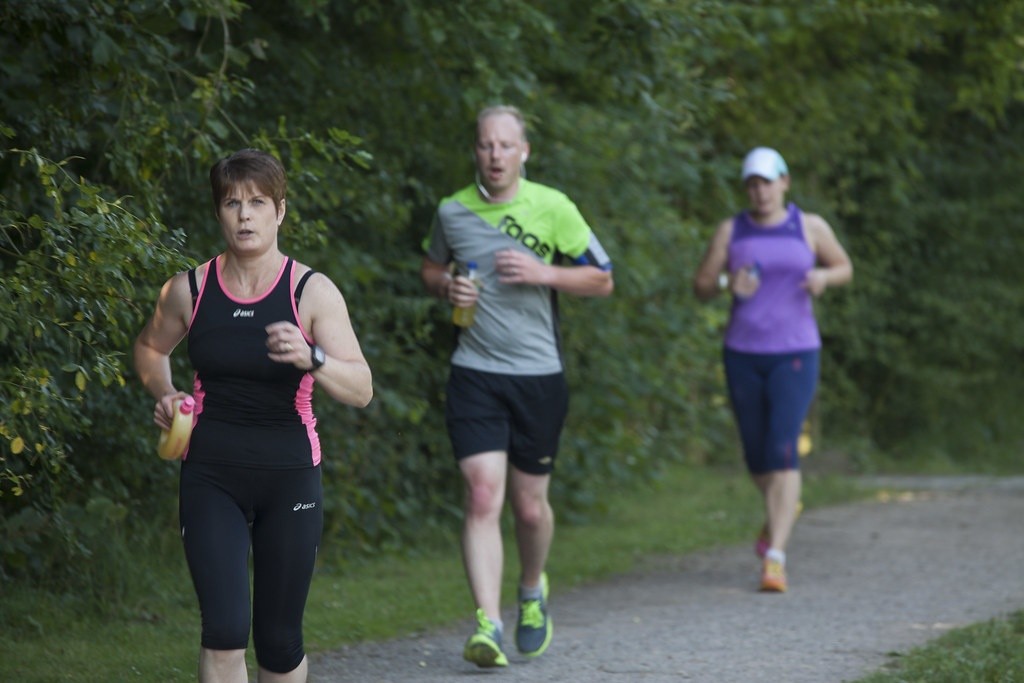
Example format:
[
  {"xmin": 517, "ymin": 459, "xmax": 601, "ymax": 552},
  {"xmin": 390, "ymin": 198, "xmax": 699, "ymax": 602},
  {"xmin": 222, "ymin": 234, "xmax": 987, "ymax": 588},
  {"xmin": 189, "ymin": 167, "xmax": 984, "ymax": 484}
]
[{"xmin": 285, "ymin": 342, "xmax": 292, "ymax": 352}]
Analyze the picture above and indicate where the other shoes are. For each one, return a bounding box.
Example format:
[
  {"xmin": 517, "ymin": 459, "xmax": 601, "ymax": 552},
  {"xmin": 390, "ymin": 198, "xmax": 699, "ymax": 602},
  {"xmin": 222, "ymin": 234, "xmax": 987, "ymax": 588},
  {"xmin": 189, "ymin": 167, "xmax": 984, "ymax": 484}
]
[
  {"xmin": 755, "ymin": 500, "xmax": 804, "ymax": 557},
  {"xmin": 761, "ymin": 560, "xmax": 786, "ymax": 592}
]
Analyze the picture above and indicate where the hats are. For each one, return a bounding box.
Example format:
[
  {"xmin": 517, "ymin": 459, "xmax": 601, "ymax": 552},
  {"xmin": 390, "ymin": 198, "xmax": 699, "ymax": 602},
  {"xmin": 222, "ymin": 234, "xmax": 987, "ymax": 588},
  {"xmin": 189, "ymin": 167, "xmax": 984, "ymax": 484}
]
[{"xmin": 741, "ymin": 146, "xmax": 787, "ymax": 186}]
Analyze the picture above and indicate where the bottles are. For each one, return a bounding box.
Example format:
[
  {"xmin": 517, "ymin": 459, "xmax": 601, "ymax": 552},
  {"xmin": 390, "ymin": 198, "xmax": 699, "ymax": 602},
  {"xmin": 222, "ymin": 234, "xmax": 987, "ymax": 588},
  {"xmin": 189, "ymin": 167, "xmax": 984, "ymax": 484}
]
[
  {"xmin": 452, "ymin": 261, "xmax": 479, "ymax": 328},
  {"xmin": 156, "ymin": 396, "xmax": 194, "ymax": 461},
  {"xmin": 747, "ymin": 260, "xmax": 762, "ymax": 282}
]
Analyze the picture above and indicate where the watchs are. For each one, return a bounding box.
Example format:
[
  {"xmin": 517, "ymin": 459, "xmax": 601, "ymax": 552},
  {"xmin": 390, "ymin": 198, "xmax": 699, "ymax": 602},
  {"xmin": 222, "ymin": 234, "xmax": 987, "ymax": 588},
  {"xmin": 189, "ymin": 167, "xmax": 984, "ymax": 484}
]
[{"xmin": 303, "ymin": 345, "xmax": 326, "ymax": 373}]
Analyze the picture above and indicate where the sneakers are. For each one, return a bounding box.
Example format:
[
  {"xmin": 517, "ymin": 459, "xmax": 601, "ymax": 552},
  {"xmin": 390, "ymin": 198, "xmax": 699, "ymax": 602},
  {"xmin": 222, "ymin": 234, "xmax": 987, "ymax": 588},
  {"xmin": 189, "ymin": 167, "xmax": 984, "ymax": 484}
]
[
  {"xmin": 516, "ymin": 569, "xmax": 552, "ymax": 657},
  {"xmin": 464, "ymin": 608, "xmax": 509, "ymax": 666}
]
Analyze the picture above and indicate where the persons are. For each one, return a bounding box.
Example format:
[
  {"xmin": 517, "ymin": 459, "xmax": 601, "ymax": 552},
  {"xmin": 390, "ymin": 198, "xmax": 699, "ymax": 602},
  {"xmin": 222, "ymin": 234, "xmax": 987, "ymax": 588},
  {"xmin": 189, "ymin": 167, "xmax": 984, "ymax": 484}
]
[
  {"xmin": 693, "ymin": 146, "xmax": 852, "ymax": 593},
  {"xmin": 132, "ymin": 148, "xmax": 375, "ymax": 683},
  {"xmin": 420, "ymin": 107, "xmax": 616, "ymax": 670}
]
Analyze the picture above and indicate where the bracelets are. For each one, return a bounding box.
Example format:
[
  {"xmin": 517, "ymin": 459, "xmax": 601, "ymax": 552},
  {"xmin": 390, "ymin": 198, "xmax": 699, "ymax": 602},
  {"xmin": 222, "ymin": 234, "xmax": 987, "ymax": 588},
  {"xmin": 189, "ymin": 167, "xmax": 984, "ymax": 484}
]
[{"xmin": 716, "ymin": 271, "xmax": 729, "ymax": 293}]
